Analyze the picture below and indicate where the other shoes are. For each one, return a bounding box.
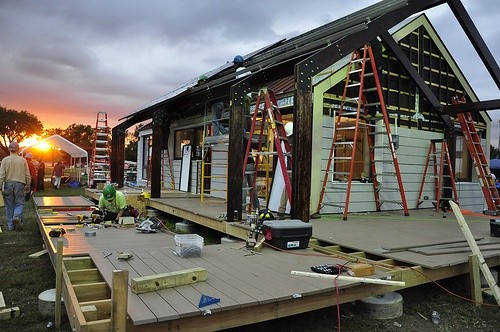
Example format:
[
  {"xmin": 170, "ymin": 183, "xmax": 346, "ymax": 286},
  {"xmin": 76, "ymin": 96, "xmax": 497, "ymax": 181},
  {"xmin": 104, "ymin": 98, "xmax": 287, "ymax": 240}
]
[{"xmin": 13, "ymin": 216, "xmax": 23, "ymax": 231}]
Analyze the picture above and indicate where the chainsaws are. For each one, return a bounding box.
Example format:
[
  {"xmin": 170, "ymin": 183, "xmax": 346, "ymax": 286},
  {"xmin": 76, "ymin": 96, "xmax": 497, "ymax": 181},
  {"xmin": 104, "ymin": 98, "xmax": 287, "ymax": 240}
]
[{"xmin": 137, "ymin": 217, "xmax": 165, "ymax": 233}]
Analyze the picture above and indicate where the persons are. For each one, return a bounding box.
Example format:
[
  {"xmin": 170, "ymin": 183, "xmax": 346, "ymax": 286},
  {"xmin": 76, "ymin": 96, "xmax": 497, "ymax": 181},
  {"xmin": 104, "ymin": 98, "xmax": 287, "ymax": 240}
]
[
  {"xmin": 52, "ymin": 159, "xmax": 65, "ymax": 189},
  {"xmin": 0, "ymin": 142, "xmax": 31, "ymax": 230},
  {"xmin": 81, "ymin": 161, "xmax": 86, "ymax": 168},
  {"xmin": 277, "ymin": 122, "xmax": 293, "ymax": 221},
  {"xmin": 187, "ymin": 75, "xmax": 219, "ymax": 135},
  {"xmin": 232, "ymin": 55, "xmax": 252, "ymax": 132},
  {"xmin": 92, "ymin": 184, "xmax": 127, "ymax": 224},
  {"xmin": 490, "ymin": 170, "xmax": 496, "ymax": 180},
  {"xmin": 355, "ymin": 38, "xmax": 386, "ymax": 118},
  {"xmin": 242, "ymin": 129, "xmax": 260, "ymax": 213},
  {"xmin": 25, "ymin": 153, "xmax": 45, "ymax": 202}
]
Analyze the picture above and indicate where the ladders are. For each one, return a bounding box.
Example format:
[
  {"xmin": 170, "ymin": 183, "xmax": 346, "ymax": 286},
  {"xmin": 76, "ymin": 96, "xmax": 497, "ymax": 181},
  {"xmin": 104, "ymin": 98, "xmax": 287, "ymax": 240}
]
[
  {"xmin": 242, "ymin": 86, "xmax": 293, "ymax": 213},
  {"xmin": 146, "ymin": 147, "xmax": 175, "ymax": 190},
  {"xmin": 316, "ymin": 42, "xmax": 411, "ymax": 220},
  {"xmin": 87, "ymin": 111, "xmax": 112, "ymax": 188},
  {"xmin": 453, "ymin": 94, "xmax": 500, "ymax": 216},
  {"xmin": 416, "ymin": 138, "xmax": 458, "ymax": 212}
]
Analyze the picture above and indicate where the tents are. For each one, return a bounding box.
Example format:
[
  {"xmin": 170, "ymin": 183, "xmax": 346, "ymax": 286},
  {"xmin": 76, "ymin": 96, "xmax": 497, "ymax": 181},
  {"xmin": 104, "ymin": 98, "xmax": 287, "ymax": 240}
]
[{"xmin": 20, "ymin": 135, "xmax": 89, "ymax": 187}]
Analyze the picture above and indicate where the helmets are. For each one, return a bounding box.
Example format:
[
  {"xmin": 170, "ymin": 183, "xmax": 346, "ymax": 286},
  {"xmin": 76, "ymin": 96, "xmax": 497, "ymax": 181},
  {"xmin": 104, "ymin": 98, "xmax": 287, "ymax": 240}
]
[
  {"xmin": 25, "ymin": 153, "xmax": 32, "ymax": 159},
  {"xmin": 233, "ymin": 55, "xmax": 245, "ymax": 62},
  {"xmin": 491, "ymin": 174, "xmax": 496, "ymax": 180},
  {"xmin": 103, "ymin": 184, "xmax": 116, "ymax": 200},
  {"xmin": 197, "ymin": 76, "xmax": 208, "ymax": 84},
  {"xmin": 8, "ymin": 142, "xmax": 19, "ymax": 150}
]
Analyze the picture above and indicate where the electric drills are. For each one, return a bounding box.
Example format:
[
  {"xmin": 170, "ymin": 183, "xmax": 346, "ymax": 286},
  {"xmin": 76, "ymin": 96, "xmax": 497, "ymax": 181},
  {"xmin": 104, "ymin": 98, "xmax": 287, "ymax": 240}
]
[{"xmin": 75, "ymin": 215, "xmax": 89, "ymax": 222}]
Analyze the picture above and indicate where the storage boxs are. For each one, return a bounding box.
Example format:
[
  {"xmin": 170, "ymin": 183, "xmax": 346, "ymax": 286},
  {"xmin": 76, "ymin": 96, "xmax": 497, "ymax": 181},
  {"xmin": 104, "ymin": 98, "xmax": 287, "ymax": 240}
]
[
  {"xmin": 261, "ymin": 219, "xmax": 312, "ymax": 251},
  {"xmin": 174, "ymin": 234, "xmax": 204, "ymax": 259}
]
